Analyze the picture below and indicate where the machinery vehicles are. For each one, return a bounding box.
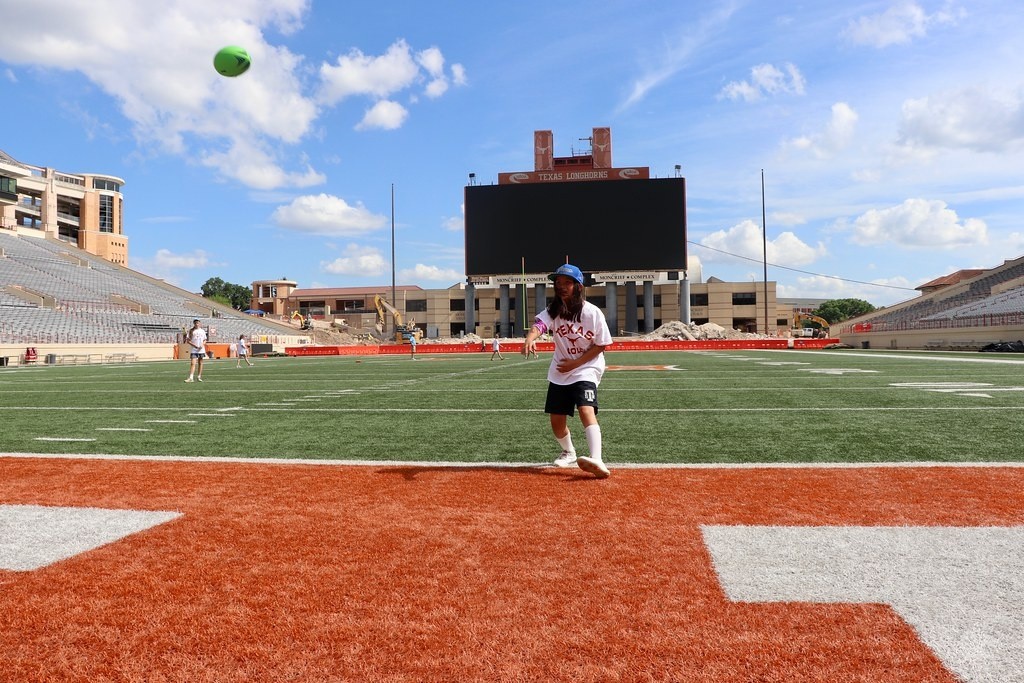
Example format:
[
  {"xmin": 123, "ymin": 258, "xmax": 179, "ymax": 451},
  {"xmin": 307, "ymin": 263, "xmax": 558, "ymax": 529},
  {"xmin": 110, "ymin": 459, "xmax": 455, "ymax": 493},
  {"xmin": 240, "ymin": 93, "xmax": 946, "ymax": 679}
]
[
  {"xmin": 792, "ymin": 311, "xmax": 829, "ymax": 338},
  {"xmin": 374, "ymin": 293, "xmax": 423, "ymax": 344},
  {"xmin": 291, "ymin": 310, "xmax": 313, "ymax": 330}
]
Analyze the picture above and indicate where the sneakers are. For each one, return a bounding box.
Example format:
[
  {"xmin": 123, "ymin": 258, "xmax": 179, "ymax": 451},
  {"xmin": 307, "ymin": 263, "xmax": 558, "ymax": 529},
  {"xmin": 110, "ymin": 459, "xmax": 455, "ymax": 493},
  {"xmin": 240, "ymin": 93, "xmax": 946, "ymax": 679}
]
[
  {"xmin": 554, "ymin": 451, "xmax": 577, "ymax": 468},
  {"xmin": 576, "ymin": 456, "xmax": 610, "ymax": 479}
]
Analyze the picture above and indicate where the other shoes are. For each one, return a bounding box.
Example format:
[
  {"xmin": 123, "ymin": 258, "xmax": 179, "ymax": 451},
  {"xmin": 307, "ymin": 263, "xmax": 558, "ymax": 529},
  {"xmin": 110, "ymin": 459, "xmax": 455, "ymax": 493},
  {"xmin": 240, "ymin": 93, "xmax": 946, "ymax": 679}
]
[
  {"xmin": 411, "ymin": 358, "xmax": 415, "ymax": 360},
  {"xmin": 501, "ymin": 358, "xmax": 505, "ymax": 361},
  {"xmin": 248, "ymin": 363, "xmax": 254, "ymax": 366},
  {"xmin": 184, "ymin": 378, "xmax": 194, "ymax": 383},
  {"xmin": 236, "ymin": 365, "xmax": 242, "ymax": 368},
  {"xmin": 491, "ymin": 359, "xmax": 494, "ymax": 361},
  {"xmin": 197, "ymin": 378, "xmax": 202, "ymax": 382}
]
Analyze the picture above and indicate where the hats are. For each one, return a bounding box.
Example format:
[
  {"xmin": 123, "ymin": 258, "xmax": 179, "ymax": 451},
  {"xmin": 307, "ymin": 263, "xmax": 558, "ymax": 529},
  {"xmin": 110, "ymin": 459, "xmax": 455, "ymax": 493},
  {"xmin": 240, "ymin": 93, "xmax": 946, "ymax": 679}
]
[{"xmin": 548, "ymin": 264, "xmax": 584, "ymax": 284}]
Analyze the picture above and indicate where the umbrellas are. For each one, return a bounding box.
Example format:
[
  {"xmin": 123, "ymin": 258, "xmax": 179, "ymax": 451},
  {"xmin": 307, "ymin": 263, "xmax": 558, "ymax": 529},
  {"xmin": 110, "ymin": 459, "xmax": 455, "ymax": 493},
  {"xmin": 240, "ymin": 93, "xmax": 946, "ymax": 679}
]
[{"xmin": 243, "ymin": 309, "xmax": 264, "ymax": 315}]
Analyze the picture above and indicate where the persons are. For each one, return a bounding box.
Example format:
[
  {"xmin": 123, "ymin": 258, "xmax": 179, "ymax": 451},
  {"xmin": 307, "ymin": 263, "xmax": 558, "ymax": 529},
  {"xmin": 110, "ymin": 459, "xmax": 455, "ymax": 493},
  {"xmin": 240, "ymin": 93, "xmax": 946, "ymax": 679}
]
[
  {"xmin": 523, "ymin": 334, "xmax": 538, "ymax": 359},
  {"xmin": 522, "ymin": 264, "xmax": 613, "ymax": 478},
  {"xmin": 491, "ymin": 334, "xmax": 504, "ymax": 361},
  {"xmin": 236, "ymin": 334, "xmax": 254, "ymax": 367},
  {"xmin": 184, "ymin": 319, "xmax": 207, "ymax": 383},
  {"xmin": 481, "ymin": 340, "xmax": 486, "ymax": 352},
  {"xmin": 410, "ymin": 334, "xmax": 418, "ymax": 360}
]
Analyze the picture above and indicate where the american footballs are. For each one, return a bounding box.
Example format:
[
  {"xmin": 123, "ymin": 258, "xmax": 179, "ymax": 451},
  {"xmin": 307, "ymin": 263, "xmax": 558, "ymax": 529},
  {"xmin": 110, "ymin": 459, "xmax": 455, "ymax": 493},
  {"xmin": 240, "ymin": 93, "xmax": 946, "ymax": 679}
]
[{"xmin": 213, "ymin": 46, "xmax": 252, "ymax": 78}]
